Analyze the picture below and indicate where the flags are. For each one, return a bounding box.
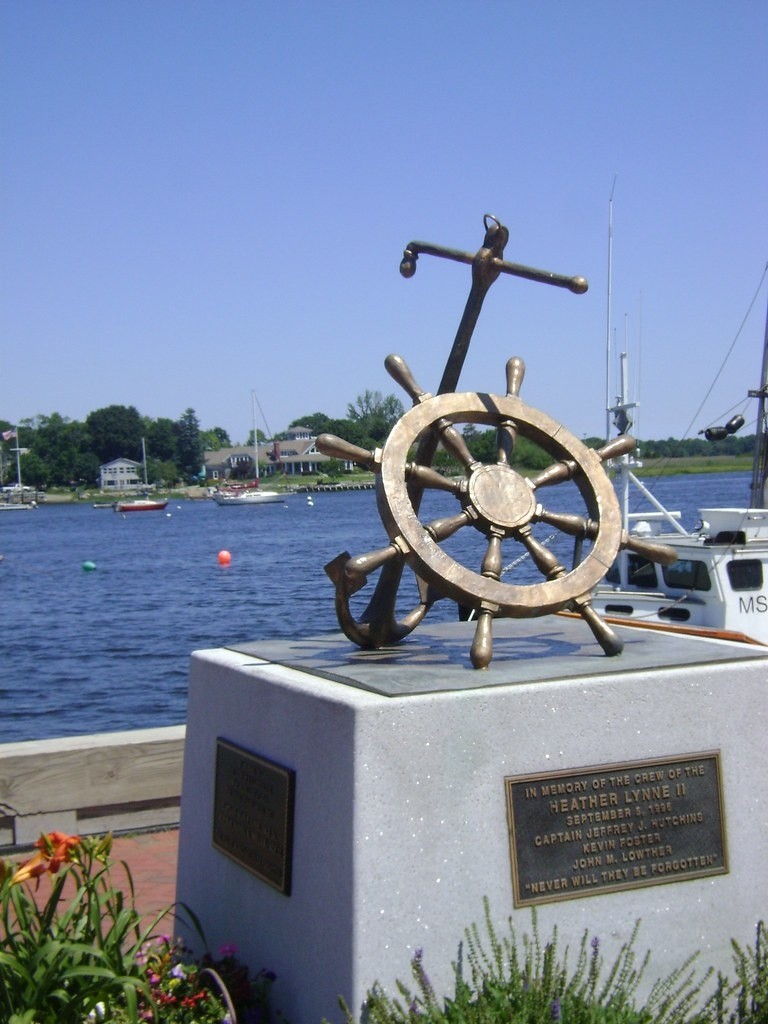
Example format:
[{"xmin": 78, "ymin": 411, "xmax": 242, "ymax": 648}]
[{"xmin": 2, "ymin": 429, "xmax": 17, "ymax": 441}]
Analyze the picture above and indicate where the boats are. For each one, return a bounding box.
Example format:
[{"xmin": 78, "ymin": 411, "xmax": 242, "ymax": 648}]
[
  {"xmin": 212, "ymin": 490, "xmax": 297, "ymax": 506},
  {"xmin": 111, "ymin": 499, "xmax": 170, "ymax": 512}
]
